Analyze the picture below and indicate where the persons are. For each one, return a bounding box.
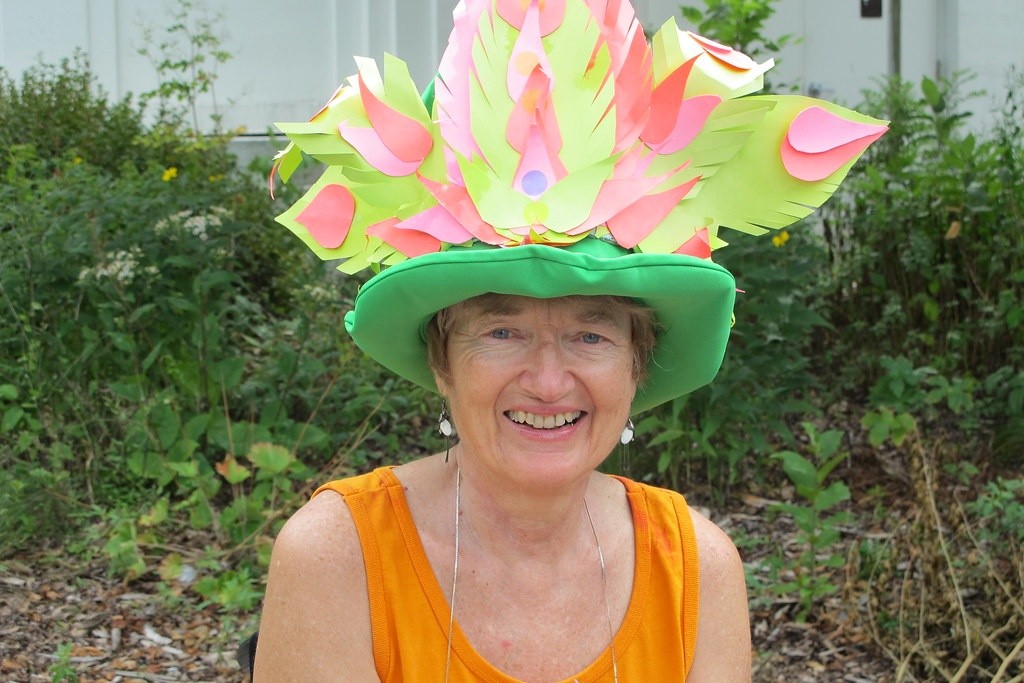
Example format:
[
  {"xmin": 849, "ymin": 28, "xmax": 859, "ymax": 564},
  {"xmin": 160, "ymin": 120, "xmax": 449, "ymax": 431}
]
[{"xmin": 253, "ymin": 292, "xmax": 753, "ymax": 683}]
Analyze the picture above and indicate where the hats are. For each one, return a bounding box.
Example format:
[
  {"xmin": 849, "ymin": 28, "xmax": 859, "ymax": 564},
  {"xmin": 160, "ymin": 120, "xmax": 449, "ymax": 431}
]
[{"xmin": 269, "ymin": 0, "xmax": 892, "ymax": 418}]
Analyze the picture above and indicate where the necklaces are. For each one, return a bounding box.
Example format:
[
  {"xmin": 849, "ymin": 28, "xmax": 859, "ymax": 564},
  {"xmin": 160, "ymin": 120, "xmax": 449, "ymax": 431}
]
[{"xmin": 445, "ymin": 465, "xmax": 619, "ymax": 682}]
